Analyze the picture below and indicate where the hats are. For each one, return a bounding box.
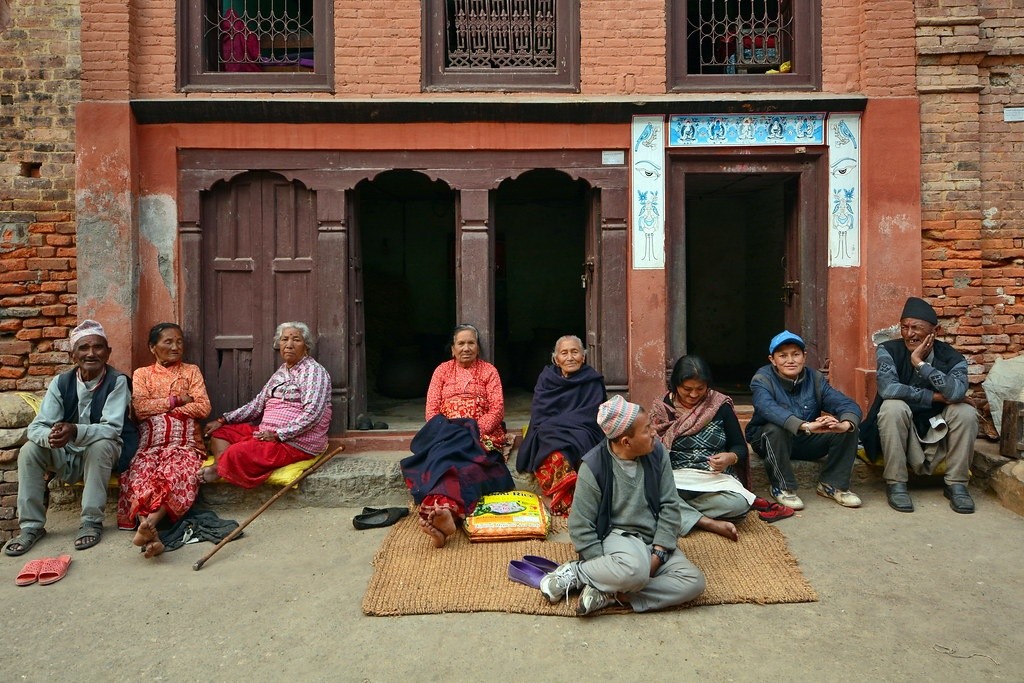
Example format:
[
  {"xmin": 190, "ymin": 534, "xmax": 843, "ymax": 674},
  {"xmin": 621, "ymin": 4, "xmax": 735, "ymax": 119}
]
[
  {"xmin": 597, "ymin": 395, "xmax": 639, "ymax": 438},
  {"xmin": 900, "ymin": 297, "xmax": 937, "ymax": 325},
  {"xmin": 769, "ymin": 330, "xmax": 805, "ymax": 356},
  {"xmin": 69, "ymin": 320, "xmax": 107, "ymax": 351}
]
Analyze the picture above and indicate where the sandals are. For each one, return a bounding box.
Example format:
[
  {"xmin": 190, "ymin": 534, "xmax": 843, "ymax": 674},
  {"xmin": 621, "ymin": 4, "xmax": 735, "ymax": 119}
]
[
  {"xmin": 5, "ymin": 527, "xmax": 46, "ymax": 556},
  {"xmin": 74, "ymin": 521, "xmax": 103, "ymax": 549}
]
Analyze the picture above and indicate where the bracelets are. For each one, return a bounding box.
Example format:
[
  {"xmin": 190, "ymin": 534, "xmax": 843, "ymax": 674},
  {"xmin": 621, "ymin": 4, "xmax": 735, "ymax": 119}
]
[
  {"xmin": 805, "ymin": 421, "xmax": 810, "ymax": 436},
  {"xmin": 916, "ymin": 361, "xmax": 926, "ymax": 373},
  {"xmin": 216, "ymin": 418, "xmax": 224, "ymax": 425}
]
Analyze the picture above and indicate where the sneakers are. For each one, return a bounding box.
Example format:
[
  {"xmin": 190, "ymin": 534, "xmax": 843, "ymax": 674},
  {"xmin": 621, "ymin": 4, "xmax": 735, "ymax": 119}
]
[
  {"xmin": 816, "ymin": 482, "xmax": 861, "ymax": 506},
  {"xmin": 769, "ymin": 485, "xmax": 804, "ymax": 509},
  {"xmin": 576, "ymin": 585, "xmax": 624, "ymax": 615},
  {"xmin": 540, "ymin": 560, "xmax": 577, "ymax": 605}
]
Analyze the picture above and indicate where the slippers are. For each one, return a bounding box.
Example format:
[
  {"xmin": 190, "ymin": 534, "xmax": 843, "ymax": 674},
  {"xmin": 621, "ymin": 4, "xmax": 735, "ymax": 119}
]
[
  {"xmin": 39, "ymin": 554, "xmax": 71, "ymax": 585},
  {"xmin": 759, "ymin": 504, "xmax": 794, "ymax": 522},
  {"xmin": 15, "ymin": 559, "xmax": 44, "ymax": 585},
  {"xmin": 751, "ymin": 498, "xmax": 777, "ymax": 511}
]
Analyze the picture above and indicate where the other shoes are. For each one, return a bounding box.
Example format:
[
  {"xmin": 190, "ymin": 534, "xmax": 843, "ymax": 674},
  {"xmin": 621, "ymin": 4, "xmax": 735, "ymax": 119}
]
[
  {"xmin": 362, "ymin": 507, "xmax": 408, "ymax": 517},
  {"xmin": 522, "ymin": 556, "xmax": 559, "ymax": 572},
  {"xmin": 375, "ymin": 421, "xmax": 389, "ymax": 430},
  {"xmin": 508, "ymin": 560, "xmax": 547, "ymax": 589},
  {"xmin": 353, "ymin": 508, "xmax": 401, "ymax": 529},
  {"xmin": 358, "ymin": 416, "xmax": 374, "ymax": 430}
]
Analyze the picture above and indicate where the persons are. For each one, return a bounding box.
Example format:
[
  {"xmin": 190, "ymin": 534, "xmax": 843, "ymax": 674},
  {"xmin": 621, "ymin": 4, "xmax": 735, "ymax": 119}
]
[
  {"xmin": 744, "ymin": 330, "xmax": 865, "ymax": 510},
  {"xmin": 860, "ymin": 295, "xmax": 979, "ymax": 514},
  {"xmin": 540, "ymin": 394, "xmax": 705, "ymax": 617},
  {"xmin": 130, "ymin": 322, "xmax": 213, "ymax": 558},
  {"xmin": 649, "ymin": 356, "xmax": 754, "ymax": 541},
  {"xmin": 532, "ymin": 334, "xmax": 608, "ymax": 517},
  {"xmin": 5, "ymin": 320, "xmax": 139, "ymax": 553},
  {"xmin": 418, "ymin": 322, "xmax": 507, "ymax": 548},
  {"xmin": 195, "ymin": 321, "xmax": 333, "ymax": 485}
]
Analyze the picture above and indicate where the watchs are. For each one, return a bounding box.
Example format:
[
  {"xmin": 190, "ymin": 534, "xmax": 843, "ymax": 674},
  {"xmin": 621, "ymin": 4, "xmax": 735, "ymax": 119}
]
[{"xmin": 652, "ymin": 549, "xmax": 669, "ymax": 564}]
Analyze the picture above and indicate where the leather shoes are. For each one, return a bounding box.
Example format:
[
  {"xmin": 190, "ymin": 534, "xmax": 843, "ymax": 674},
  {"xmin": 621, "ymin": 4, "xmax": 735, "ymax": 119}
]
[
  {"xmin": 886, "ymin": 483, "xmax": 914, "ymax": 512},
  {"xmin": 944, "ymin": 484, "xmax": 975, "ymax": 513}
]
